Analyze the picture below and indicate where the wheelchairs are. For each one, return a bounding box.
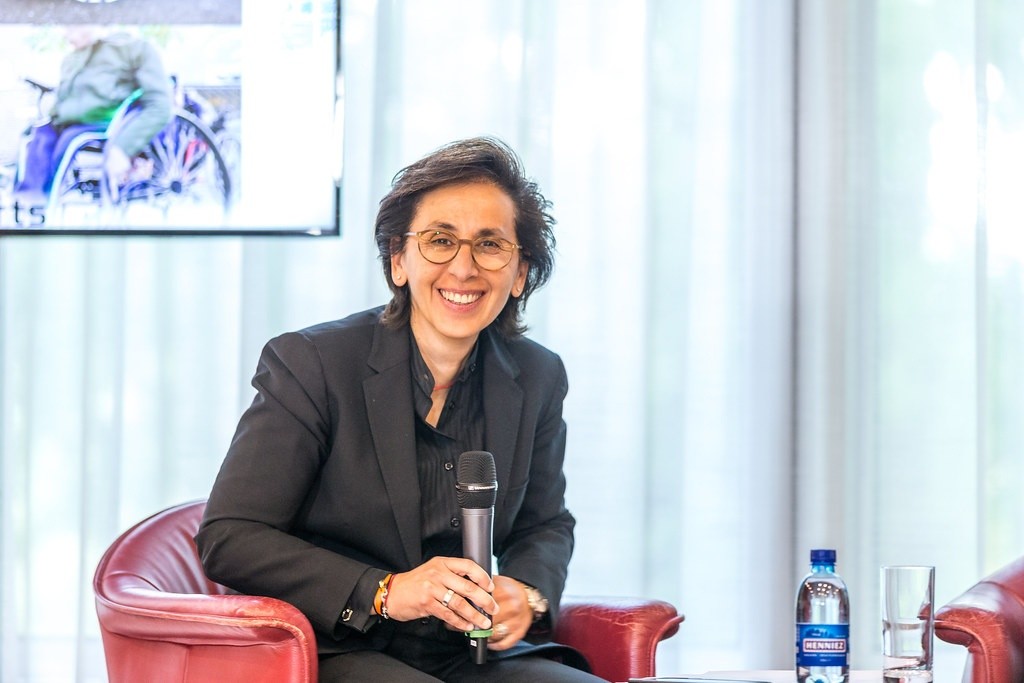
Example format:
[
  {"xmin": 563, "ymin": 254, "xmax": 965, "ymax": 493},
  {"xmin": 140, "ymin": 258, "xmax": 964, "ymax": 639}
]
[{"xmin": 0, "ymin": 74, "xmax": 232, "ymax": 224}]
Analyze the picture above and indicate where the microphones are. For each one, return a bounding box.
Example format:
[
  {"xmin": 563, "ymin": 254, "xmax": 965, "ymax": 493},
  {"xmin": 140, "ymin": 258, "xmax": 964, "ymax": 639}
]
[{"xmin": 456, "ymin": 450, "xmax": 499, "ymax": 666}]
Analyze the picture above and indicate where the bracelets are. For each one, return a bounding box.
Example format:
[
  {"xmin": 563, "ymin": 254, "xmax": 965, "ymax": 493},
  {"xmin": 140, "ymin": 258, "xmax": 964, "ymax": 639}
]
[{"xmin": 372, "ymin": 572, "xmax": 395, "ymax": 621}]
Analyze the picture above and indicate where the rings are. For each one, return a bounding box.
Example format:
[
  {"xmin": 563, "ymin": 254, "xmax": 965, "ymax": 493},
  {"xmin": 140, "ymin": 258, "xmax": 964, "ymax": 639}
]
[{"xmin": 439, "ymin": 588, "xmax": 455, "ymax": 607}]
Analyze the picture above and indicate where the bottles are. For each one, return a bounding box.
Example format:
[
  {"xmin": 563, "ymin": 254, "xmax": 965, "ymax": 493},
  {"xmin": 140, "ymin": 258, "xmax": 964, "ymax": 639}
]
[{"xmin": 796, "ymin": 549, "xmax": 850, "ymax": 683}]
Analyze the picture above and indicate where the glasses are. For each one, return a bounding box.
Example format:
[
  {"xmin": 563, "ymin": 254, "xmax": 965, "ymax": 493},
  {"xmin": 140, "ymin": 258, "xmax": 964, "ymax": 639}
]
[{"xmin": 405, "ymin": 228, "xmax": 524, "ymax": 272}]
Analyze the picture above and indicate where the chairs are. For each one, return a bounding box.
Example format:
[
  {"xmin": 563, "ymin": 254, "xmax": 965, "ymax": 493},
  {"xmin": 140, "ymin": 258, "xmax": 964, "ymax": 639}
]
[
  {"xmin": 933, "ymin": 555, "xmax": 1024, "ymax": 683},
  {"xmin": 93, "ymin": 497, "xmax": 686, "ymax": 683}
]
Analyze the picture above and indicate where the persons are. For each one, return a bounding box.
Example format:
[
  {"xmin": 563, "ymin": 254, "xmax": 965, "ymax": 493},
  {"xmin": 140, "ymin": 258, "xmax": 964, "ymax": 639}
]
[
  {"xmin": 13, "ymin": 22, "xmax": 184, "ymax": 221},
  {"xmin": 189, "ymin": 137, "xmax": 617, "ymax": 683}
]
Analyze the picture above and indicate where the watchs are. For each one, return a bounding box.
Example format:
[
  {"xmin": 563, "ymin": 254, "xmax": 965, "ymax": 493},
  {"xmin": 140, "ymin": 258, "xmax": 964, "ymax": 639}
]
[{"xmin": 523, "ymin": 582, "xmax": 549, "ymax": 623}]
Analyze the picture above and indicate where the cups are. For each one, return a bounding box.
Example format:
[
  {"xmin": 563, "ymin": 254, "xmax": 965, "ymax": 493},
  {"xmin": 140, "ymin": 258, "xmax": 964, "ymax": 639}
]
[{"xmin": 879, "ymin": 565, "xmax": 934, "ymax": 683}]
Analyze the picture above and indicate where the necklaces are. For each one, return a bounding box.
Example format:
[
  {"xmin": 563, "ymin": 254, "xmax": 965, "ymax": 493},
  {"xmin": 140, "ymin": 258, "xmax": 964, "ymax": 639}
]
[{"xmin": 426, "ymin": 375, "xmax": 457, "ymax": 392}]
